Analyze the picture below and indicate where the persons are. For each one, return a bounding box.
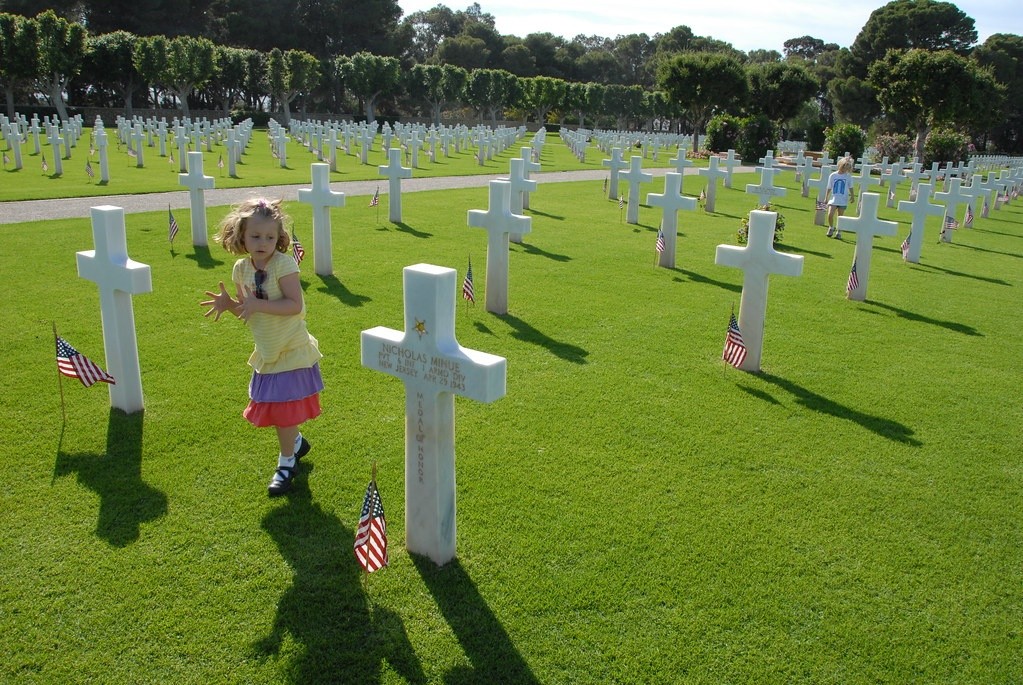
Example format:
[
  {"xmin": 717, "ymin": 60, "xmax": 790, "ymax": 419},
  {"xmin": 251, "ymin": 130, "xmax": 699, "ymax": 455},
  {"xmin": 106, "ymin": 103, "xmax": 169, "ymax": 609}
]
[{"xmin": 198, "ymin": 194, "xmax": 325, "ymax": 495}]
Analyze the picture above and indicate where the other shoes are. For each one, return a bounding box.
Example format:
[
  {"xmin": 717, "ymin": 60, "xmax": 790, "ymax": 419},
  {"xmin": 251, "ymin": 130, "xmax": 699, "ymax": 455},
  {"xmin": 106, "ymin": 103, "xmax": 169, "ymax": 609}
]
[
  {"xmin": 294, "ymin": 437, "xmax": 310, "ymax": 463},
  {"xmin": 268, "ymin": 460, "xmax": 299, "ymax": 493}
]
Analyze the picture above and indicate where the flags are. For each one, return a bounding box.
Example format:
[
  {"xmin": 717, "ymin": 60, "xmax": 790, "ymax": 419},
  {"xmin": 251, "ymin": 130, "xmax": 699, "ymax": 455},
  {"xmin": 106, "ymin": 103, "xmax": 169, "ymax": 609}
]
[
  {"xmin": 462, "ymin": 260, "xmax": 476, "ymax": 306},
  {"xmin": 945, "ymin": 215, "xmax": 960, "ymax": 231},
  {"xmin": 1, "ymin": 105, "xmax": 736, "ymax": 188},
  {"xmin": 846, "ymin": 257, "xmax": 859, "ymax": 292},
  {"xmin": 982, "ymin": 200, "xmax": 990, "ymax": 217},
  {"xmin": 697, "ymin": 188, "xmax": 705, "ymax": 203},
  {"xmin": 368, "ymin": 188, "xmax": 379, "ymax": 208},
  {"xmin": 965, "ymin": 204, "xmax": 974, "ymax": 225},
  {"xmin": 881, "ymin": 154, "xmax": 1023, "ymax": 208},
  {"xmin": 801, "ymin": 181, "xmax": 806, "ymax": 195},
  {"xmin": 619, "ymin": 196, "xmax": 624, "ymax": 210},
  {"xmin": 900, "ymin": 232, "xmax": 912, "ymax": 261},
  {"xmin": 814, "ymin": 200, "xmax": 828, "ymax": 213},
  {"xmin": 602, "ymin": 178, "xmax": 608, "ymax": 192},
  {"xmin": 655, "ymin": 228, "xmax": 667, "ymax": 254},
  {"xmin": 722, "ymin": 312, "xmax": 748, "ymax": 368},
  {"xmin": 352, "ymin": 478, "xmax": 391, "ymax": 574},
  {"xmin": 54, "ymin": 337, "xmax": 117, "ymax": 388},
  {"xmin": 292, "ymin": 233, "xmax": 305, "ymax": 267},
  {"xmin": 168, "ymin": 210, "xmax": 179, "ymax": 244}
]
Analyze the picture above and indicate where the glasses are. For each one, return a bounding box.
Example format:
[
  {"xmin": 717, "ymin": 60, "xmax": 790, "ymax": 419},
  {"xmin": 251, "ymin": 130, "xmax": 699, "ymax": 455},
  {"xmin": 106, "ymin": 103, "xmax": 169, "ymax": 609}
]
[{"xmin": 255, "ymin": 269, "xmax": 268, "ymax": 301}]
